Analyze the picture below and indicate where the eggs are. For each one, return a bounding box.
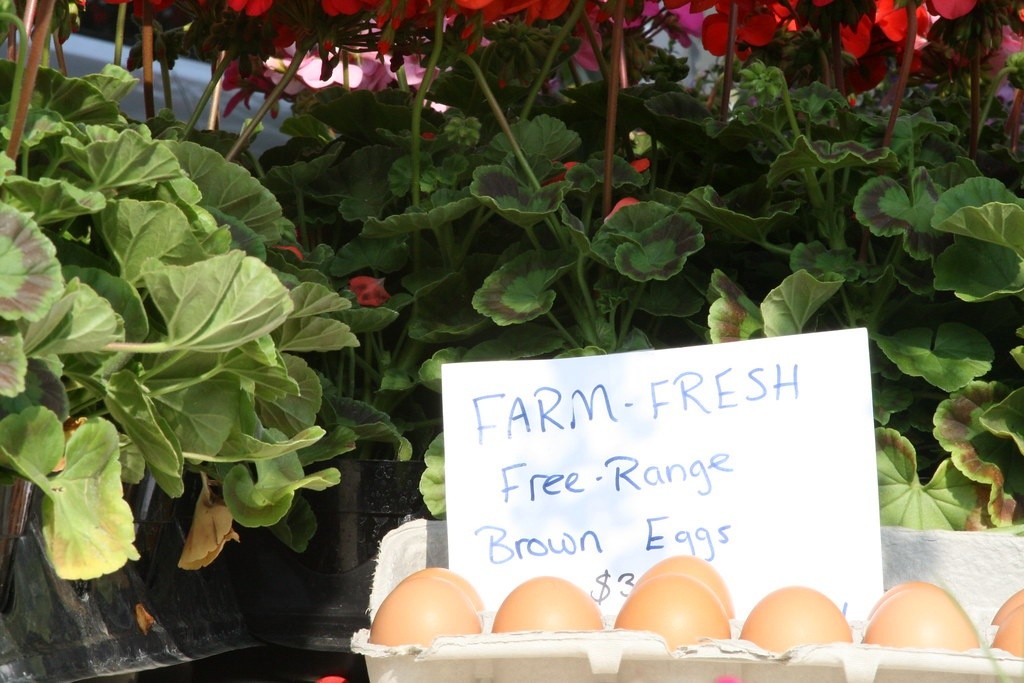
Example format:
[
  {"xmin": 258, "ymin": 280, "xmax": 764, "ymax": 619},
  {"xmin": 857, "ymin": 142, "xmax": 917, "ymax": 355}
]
[{"xmin": 368, "ymin": 556, "xmax": 1024, "ymax": 660}]
